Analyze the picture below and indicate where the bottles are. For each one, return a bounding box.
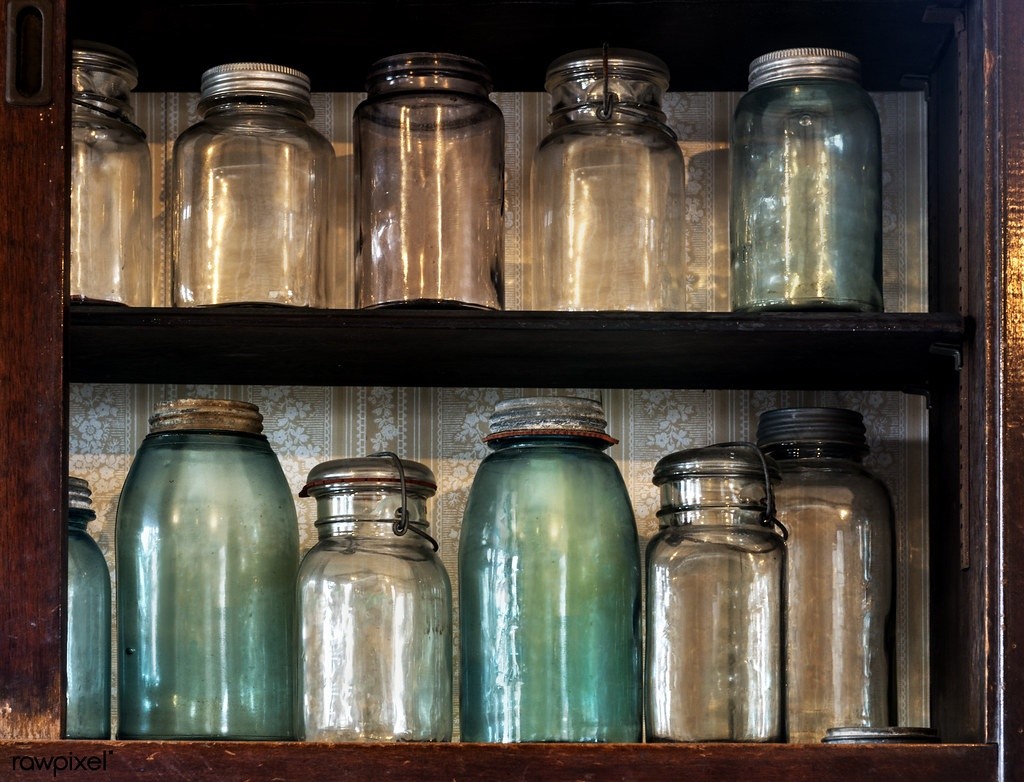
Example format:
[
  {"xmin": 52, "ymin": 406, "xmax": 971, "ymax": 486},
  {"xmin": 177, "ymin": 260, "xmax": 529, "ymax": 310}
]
[
  {"xmin": 455, "ymin": 394, "xmax": 645, "ymax": 748},
  {"xmin": 113, "ymin": 395, "xmax": 301, "ymax": 744},
  {"xmin": 65, "ymin": 45, "xmax": 156, "ymax": 307},
  {"xmin": 348, "ymin": 51, "xmax": 509, "ymax": 314},
  {"xmin": 527, "ymin": 51, "xmax": 695, "ymax": 316},
  {"xmin": 171, "ymin": 62, "xmax": 332, "ymax": 314},
  {"xmin": 57, "ymin": 471, "xmax": 117, "ymax": 740},
  {"xmin": 721, "ymin": 46, "xmax": 888, "ymax": 319},
  {"xmin": 637, "ymin": 443, "xmax": 793, "ymax": 746},
  {"xmin": 290, "ymin": 448, "xmax": 457, "ymax": 748},
  {"xmin": 749, "ymin": 407, "xmax": 901, "ymax": 743}
]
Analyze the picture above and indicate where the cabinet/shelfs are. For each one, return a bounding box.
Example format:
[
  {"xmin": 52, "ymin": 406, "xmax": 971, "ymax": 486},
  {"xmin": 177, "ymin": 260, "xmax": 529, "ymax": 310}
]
[{"xmin": 57, "ymin": 0, "xmax": 1002, "ymax": 780}]
